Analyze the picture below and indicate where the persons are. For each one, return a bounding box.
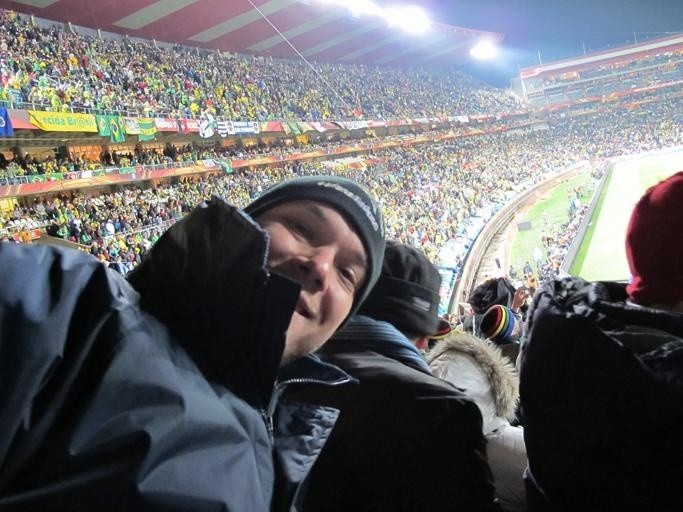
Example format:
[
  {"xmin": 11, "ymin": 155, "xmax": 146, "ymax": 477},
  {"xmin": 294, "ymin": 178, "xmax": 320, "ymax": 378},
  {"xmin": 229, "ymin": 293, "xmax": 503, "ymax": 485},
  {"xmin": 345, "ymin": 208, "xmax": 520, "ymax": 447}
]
[{"xmin": 1, "ymin": 8, "xmax": 682, "ymax": 512}]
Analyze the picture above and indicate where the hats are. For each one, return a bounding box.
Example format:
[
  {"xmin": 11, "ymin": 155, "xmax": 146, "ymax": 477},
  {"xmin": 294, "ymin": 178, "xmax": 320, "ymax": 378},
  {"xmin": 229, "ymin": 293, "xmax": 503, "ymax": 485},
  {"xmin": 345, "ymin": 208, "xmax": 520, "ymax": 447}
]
[
  {"xmin": 242, "ymin": 176, "xmax": 385, "ymax": 333},
  {"xmin": 356, "ymin": 242, "xmax": 441, "ymax": 337},
  {"xmin": 625, "ymin": 172, "xmax": 683, "ymax": 304},
  {"xmin": 479, "ymin": 305, "xmax": 514, "ymax": 341}
]
